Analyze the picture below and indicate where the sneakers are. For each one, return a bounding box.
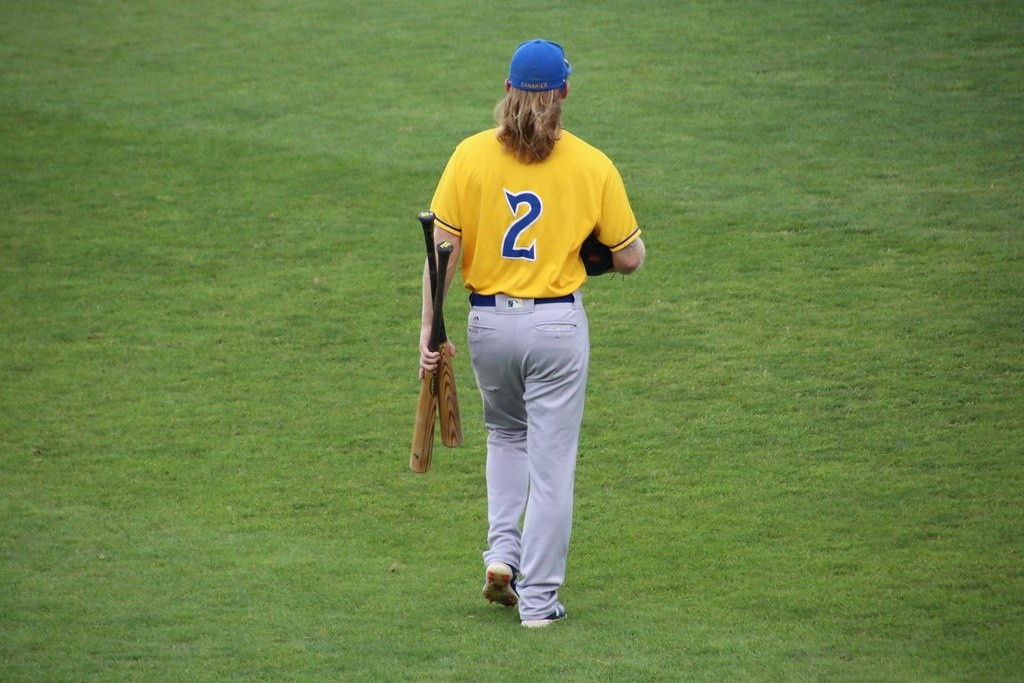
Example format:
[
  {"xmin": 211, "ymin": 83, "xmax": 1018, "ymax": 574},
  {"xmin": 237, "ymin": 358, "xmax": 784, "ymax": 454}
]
[
  {"xmin": 521, "ymin": 601, "xmax": 567, "ymax": 627},
  {"xmin": 482, "ymin": 561, "xmax": 520, "ymax": 608}
]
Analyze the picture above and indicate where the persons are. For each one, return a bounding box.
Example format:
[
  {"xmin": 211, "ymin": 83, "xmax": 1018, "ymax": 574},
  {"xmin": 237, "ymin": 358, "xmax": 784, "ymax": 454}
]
[{"xmin": 418, "ymin": 37, "xmax": 643, "ymax": 627}]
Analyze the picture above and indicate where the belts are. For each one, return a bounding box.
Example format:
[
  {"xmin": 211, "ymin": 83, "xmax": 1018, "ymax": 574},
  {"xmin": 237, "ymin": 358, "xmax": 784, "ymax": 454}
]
[{"xmin": 470, "ymin": 294, "xmax": 575, "ymax": 306}]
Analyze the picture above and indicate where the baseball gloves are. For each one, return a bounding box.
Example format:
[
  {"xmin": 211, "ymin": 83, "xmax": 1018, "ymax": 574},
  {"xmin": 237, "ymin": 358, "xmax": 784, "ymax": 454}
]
[{"xmin": 580, "ymin": 232, "xmax": 614, "ymax": 277}]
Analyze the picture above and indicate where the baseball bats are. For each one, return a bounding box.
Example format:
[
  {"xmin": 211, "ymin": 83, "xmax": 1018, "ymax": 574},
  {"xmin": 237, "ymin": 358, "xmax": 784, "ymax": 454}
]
[
  {"xmin": 407, "ymin": 239, "xmax": 454, "ymax": 474},
  {"xmin": 416, "ymin": 209, "xmax": 464, "ymax": 450}
]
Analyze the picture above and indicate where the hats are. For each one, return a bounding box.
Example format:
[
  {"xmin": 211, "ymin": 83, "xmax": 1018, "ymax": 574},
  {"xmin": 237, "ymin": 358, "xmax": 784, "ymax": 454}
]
[{"xmin": 508, "ymin": 38, "xmax": 572, "ymax": 90}]
